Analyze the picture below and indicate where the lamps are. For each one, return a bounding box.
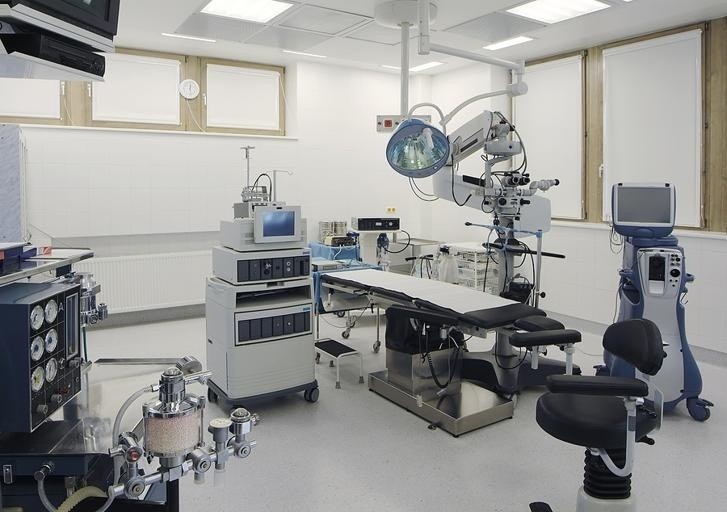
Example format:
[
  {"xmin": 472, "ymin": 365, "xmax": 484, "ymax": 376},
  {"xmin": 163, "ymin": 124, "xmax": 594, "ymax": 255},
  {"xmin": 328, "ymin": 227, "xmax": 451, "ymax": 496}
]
[{"xmin": 374, "ymin": 1, "xmax": 531, "ymax": 179}]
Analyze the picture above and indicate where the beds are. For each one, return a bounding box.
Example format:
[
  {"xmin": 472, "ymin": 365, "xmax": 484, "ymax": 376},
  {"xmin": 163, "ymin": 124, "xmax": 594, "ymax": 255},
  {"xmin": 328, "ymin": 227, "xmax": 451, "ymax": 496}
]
[{"xmin": 316, "ymin": 268, "xmax": 547, "ymax": 438}]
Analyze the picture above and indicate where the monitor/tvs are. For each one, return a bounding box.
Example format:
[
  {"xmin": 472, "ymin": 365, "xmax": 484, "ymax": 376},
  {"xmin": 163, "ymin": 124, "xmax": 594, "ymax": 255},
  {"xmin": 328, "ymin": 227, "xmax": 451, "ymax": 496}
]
[
  {"xmin": 614, "ymin": 183, "xmax": 675, "ymax": 228},
  {"xmin": 0, "ymin": 0, "xmax": 121, "ymax": 36},
  {"xmin": 254, "ymin": 205, "xmax": 302, "ymax": 243}
]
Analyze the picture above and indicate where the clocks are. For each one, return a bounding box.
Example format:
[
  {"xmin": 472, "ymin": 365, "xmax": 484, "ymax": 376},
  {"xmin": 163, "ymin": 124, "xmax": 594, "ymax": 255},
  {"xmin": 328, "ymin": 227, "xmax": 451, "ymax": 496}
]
[{"xmin": 180, "ymin": 79, "xmax": 199, "ymax": 100}]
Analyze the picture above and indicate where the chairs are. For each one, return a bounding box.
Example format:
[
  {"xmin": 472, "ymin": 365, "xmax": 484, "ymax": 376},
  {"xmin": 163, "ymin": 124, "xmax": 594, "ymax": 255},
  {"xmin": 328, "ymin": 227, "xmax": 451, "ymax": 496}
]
[{"xmin": 530, "ymin": 320, "xmax": 667, "ymax": 510}]
